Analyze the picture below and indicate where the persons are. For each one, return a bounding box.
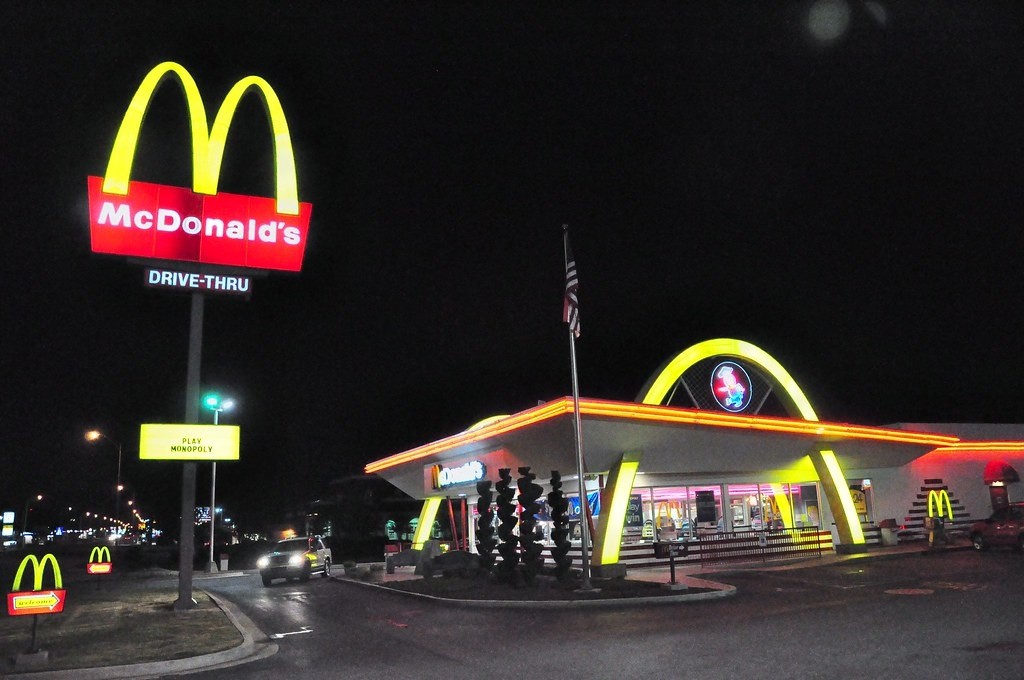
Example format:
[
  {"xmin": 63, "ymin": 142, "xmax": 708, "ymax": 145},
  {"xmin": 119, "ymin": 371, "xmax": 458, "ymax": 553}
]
[
  {"xmin": 718, "ymin": 516, "xmax": 724, "ymax": 538},
  {"xmin": 934, "ymin": 512, "xmax": 955, "ymax": 544},
  {"xmin": 311, "ymin": 535, "xmax": 321, "ymax": 551},
  {"xmin": 768, "ymin": 506, "xmax": 782, "ymax": 526}
]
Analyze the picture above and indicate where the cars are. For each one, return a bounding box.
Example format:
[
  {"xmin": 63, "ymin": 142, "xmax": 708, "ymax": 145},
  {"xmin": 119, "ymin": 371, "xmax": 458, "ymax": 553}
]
[{"xmin": 968, "ymin": 502, "xmax": 1024, "ymax": 553}]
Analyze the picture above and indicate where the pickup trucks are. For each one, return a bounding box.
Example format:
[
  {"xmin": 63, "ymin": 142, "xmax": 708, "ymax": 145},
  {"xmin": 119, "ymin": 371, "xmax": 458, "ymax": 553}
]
[{"xmin": 259, "ymin": 537, "xmax": 332, "ymax": 587}]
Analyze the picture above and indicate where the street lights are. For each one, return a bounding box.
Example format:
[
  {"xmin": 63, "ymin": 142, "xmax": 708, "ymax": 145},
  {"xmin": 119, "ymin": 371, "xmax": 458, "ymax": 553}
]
[
  {"xmin": 202, "ymin": 392, "xmax": 236, "ymax": 574},
  {"xmin": 84, "ymin": 430, "xmax": 121, "ymax": 552},
  {"xmin": 23, "ymin": 495, "xmax": 43, "ymax": 531}
]
